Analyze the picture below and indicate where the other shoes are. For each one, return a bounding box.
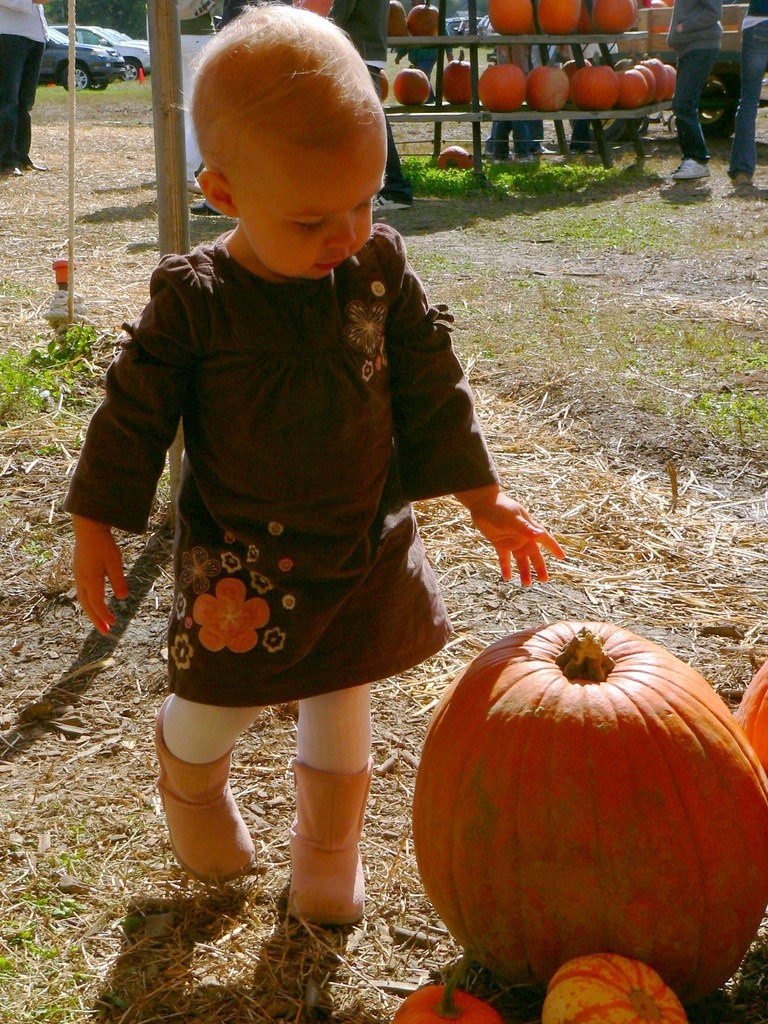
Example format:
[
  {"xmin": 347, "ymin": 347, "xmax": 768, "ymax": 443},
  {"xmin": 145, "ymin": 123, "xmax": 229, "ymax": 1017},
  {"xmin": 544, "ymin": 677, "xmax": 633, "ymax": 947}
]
[
  {"xmin": 0, "ymin": 167, "xmax": 23, "ymax": 177},
  {"xmin": 735, "ymin": 174, "xmax": 751, "ymax": 187},
  {"xmin": 486, "ymin": 145, "xmax": 557, "ymax": 164},
  {"xmin": 22, "ymin": 164, "xmax": 46, "ymax": 172},
  {"xmin": 191, "ymin": 203, "xmax": 221, "ymax": 215},
  {"xmin": 373, "ymin": 196, "xmax": 413, "ymax": 211}
]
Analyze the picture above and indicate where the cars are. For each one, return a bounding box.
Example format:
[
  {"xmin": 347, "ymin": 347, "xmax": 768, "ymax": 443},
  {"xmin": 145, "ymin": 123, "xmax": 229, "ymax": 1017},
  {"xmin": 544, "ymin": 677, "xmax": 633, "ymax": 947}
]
[
  {"xmin": 38, "ymin": 28, "xmax": 126, "ymax": 92},
  {"xmin": 45, "ymin": 25, "xmax": 151, "ymax": 83}
]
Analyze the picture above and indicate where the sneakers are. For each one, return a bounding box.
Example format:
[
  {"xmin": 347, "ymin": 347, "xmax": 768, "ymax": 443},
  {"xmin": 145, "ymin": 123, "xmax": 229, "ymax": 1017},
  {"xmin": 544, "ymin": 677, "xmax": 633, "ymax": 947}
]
[{"xmin": 673, "ymin": 159, "xmax": 710, "ymax": 180}]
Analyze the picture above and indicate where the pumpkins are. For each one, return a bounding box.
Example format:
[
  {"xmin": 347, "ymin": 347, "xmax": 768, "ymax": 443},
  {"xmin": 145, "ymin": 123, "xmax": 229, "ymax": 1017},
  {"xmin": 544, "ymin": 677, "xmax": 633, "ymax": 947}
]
[
  {"xmin": 437, "ymin": 146, "xmax": 473, "ymax": 170},
  {"xmin": 292, "ymin": 0, "xmax": 678, "ymax": 112},
  {"xmin": 386, "ymin": 622, "xmax": 768, "ymax": 1024}
]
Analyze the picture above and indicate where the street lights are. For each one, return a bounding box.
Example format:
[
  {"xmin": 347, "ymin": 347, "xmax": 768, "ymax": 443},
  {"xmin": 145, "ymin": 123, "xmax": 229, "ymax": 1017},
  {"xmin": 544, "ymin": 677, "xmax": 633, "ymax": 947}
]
[{"xmin": 139, "ymin": 68, "xmax": 145, "ymax": 85}]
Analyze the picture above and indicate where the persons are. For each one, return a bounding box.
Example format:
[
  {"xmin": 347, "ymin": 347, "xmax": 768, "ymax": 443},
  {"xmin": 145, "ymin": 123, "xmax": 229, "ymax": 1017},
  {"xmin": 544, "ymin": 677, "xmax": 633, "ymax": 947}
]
[
  {"xmin": 175, "ymin": 0, "xmax": 412, "ymax": 217},
  {"xmin": 0, "ymin": 0, "xmax": 51, "ymax": 178},
  {"xmin": 395, "ymin": 0, "xmax": 454, "ymax": 103},
  {"xmin": 65, "ymin": 4, "xmax": 564, "ymax": 927},
  {"xmin": 477, "ymin": 0, "xmax": 768, "ymax": 184}
]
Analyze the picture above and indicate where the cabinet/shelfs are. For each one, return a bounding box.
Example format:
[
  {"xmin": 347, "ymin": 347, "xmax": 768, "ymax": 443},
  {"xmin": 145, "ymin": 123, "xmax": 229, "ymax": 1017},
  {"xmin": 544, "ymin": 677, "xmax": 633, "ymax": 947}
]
[{"xmin": 385, "ymin": 30, "xmax": 673, "ymax": 122}]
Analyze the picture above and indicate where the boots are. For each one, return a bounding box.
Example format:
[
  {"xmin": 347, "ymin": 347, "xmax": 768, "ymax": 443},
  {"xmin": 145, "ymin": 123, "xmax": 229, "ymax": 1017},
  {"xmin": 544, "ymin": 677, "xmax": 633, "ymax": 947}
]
[
  {"xmin": 289, "ymin": 757, "xmax": 374, "ymax": 925},
  {"xmin": 155, "ymin": 693, "xmax": 255, "ymax": 884}
]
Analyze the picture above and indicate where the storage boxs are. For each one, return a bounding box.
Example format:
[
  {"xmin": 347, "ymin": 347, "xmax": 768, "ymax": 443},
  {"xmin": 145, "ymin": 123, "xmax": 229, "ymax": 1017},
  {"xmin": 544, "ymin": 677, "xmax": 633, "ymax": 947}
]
[{"xmin": 617, "ymin": 5, "xmax": 749, "ymax": 55}]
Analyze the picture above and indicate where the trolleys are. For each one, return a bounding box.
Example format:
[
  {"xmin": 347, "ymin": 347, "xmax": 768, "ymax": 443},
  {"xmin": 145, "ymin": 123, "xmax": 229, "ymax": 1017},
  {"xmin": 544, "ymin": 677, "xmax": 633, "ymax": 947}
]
[{"xmin": 568, "ymin": 4, "xmax": 752, "ymax": 141}]
